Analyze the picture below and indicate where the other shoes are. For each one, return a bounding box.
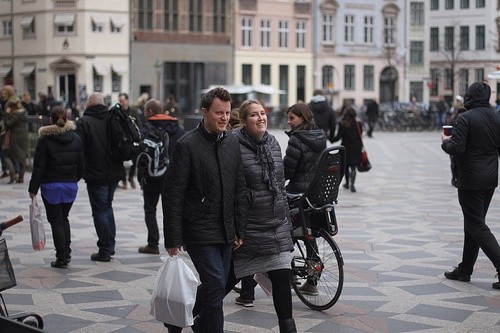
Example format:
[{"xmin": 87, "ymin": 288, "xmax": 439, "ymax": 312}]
[
  {"xmin": 9, "ymin": 176, "xmax": 15, "ymax": 184},
  {"xmin": 0, "ymin": 173, "xmax": 8, "ymax": 178},
  {"xmin": 138, "ymin": 245, "xmax": 160, "ymax": 255},
  {"xmin": 90, "ymin": 251, "xmax": 111, "ymax": 262},
  {"xmin": 343, "ymin": 184, "xmax": 349, "ymax": 188},
  {"xmin": 235, "ymin": 296, "xmax": 254, "ymax": 307},
  {"xmin": 444, "ymin": 267, "xmax": 470, "ymax": 281},
  {"xmin": 51, "ymin": 260, "xmax": 67, "ymax": 269},
  {"xmin": 351, "ymin": 187, "xmax": 356, "ymax": 191},
  {"xmin": 492, "ymin": 282, "xmax": 500, "ymax": 289},
  {"xmin": 17, "ymin": 180, "xmax": 23, "ymax": 183},
  {"xmin": 129, "ymin": 178, "xmax": 136, "ymax": 189},
  {"xmin": 295, "ymin": 281, "xmax": 318, "ymax": 295}
]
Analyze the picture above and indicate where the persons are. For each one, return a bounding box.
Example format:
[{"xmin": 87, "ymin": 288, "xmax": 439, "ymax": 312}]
[
  {"xmin": 282, "ymin": 103, "xmax": 327, "ymax": 295},
  {"xmin": 75, "ymin": 93, "xmax": 125, "ymax": 262},
  {"xmin": 440, "ymin": 81, "xmax": 500, "ymax": 289},
  {"xmin": 161, "ymin": 87, "xmax": 248, "ymax": 333},
  {"xmin": 307, "ymin": 89, "xmax": 337, "ymax": 140},
  {"xmin": 224, "ymin": 100, "xmax": 298, "ymax": 333},
  {"xmin": 28, "ymin": 107, "xmax": 82, "ymax": 267},
  {"xmin": 116, "ymin": 94, "xmax": 141, "ymax": 188},
  {"xmin": 163, "ymin": 95, "xmax": 181, "ymax": 117},
  {"xmin": 335, "ymin": 107, "xmax": 363, "ymax": 191},
  {"xmin": 365, "ymin": 98, "xmax": 379, "ymax": 136},
  {"xmin": 0, "ymin": 85, "xmax": 79, "ymax": 183},
  {"xmin": 136, "ymin": 99, "xmax": 180, "ymax": 254}
]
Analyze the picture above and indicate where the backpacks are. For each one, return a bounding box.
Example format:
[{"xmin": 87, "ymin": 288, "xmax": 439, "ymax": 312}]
[{"xmin": 137, "ymin": 123, "xmax": 169, "ymax": 175}]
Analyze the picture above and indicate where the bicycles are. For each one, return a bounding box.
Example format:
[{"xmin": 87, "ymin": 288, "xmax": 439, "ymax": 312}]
[
  {"xmin": 231, "ymin": 192, "xmax": 345, "ymax": 312},
  {"xmin": 0, "ymin": 214, "xmax": 24, "ymax": 319}
]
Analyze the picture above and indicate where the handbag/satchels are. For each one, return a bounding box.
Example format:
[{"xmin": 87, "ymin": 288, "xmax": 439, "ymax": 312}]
[
  {"xmin": 28, "ymin": 197, "xmax": 45, "ymax": 250},
  {"xmin": 357, "ymin": 157, "xmax": 371, "ymax": 171},
  {"xmin": 149, "ymin": 249, "xmax": 198, "ymax": 327},
  {"xmin": 110, "ymin": 104, "xmax": 139, "ymax": 153}
]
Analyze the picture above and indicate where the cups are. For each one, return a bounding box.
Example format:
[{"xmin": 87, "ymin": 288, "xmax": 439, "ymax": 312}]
[{"xmin": 443, "ymin": 126, "xmax": 453, "ymax": 136}]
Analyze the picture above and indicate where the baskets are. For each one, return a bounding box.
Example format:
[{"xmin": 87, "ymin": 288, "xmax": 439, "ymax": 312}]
[{"xmin": 0, "ymin": 239, "xmax": 18, "ymax": 292}]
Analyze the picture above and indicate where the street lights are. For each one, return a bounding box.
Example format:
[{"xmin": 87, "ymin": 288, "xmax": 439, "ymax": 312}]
[{"xmin": 153, "ymin": 56, "xmax": 164, "ymax": 100}]
